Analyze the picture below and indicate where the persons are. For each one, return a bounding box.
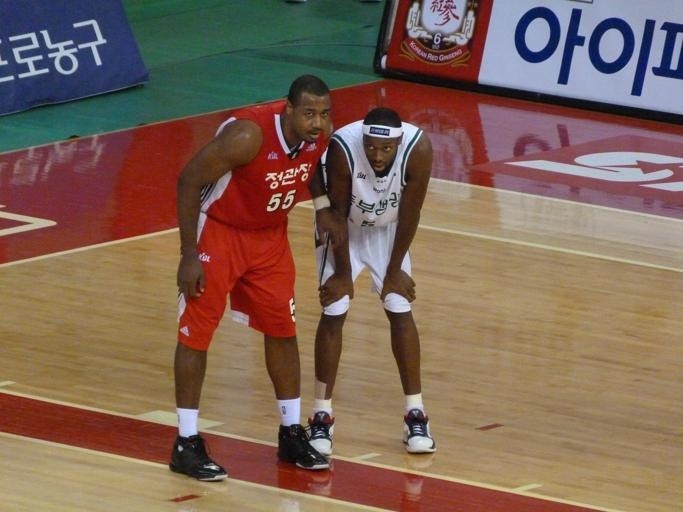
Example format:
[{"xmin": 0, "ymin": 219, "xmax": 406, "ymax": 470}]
[
  {"xmin": 308, "ymin": 108, "xmax": 437, "ymax": 456},
  {"xmin": 169, "ymin": 74, "xmax": 349, "ymax": 482}
]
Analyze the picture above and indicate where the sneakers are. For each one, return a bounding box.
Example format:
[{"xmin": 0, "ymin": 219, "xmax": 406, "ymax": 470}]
[
  {"xmin": 306, "ymin": 407, "xmax": 335, "ymax": 457},
  {"xmin": 400, "ymin": 403, "xmax": 436, "ymax": 454},
  {"xmin": 276, "ymin": 425, "xmax": 330, "ymax": 471},
  {"xmin": 168, "ymin": 434, "xmax": 227, "ymax": 481}
]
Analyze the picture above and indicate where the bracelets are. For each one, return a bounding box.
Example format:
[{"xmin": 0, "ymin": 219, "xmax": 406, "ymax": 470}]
[{"xmin": 313, "ymin": 194, "xmax": 332, "ymax": 211}]
